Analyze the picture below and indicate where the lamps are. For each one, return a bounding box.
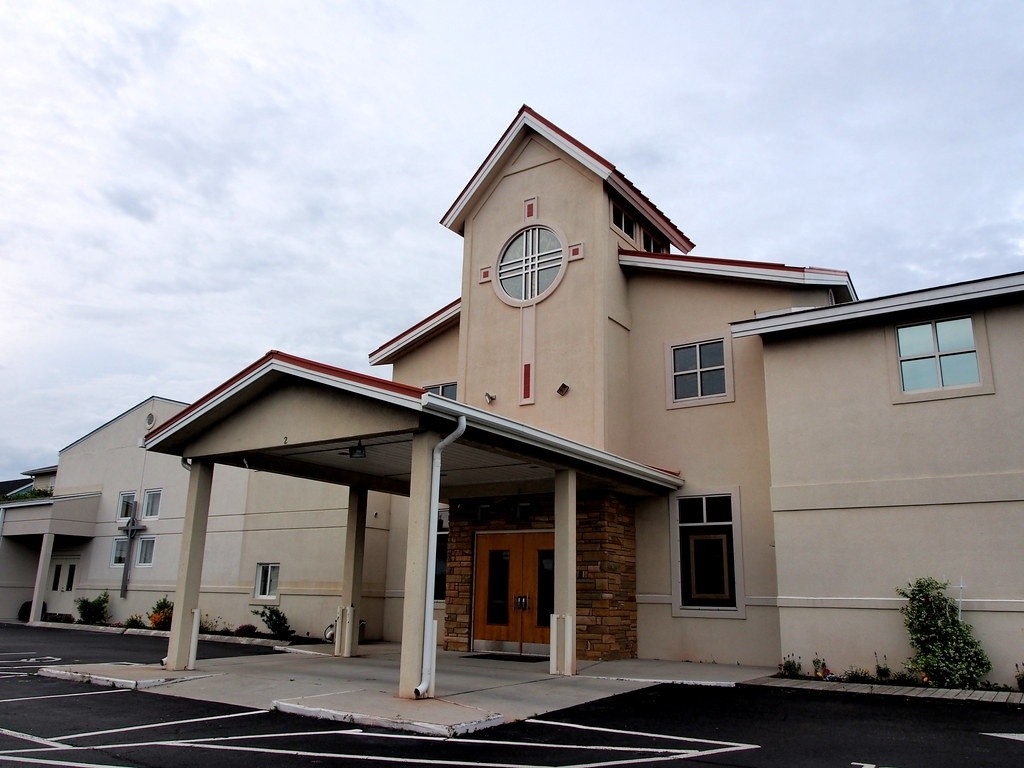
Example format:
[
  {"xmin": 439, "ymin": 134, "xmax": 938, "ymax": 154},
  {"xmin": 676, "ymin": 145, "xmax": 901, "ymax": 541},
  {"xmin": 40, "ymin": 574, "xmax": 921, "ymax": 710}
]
[
  {"xmin": 349, "ymin": 440, "xmax": 366, "ymax": 458},
  {"xmin": 485, "ymin": 392, "xmax": 496, "ymax": 403}
]
